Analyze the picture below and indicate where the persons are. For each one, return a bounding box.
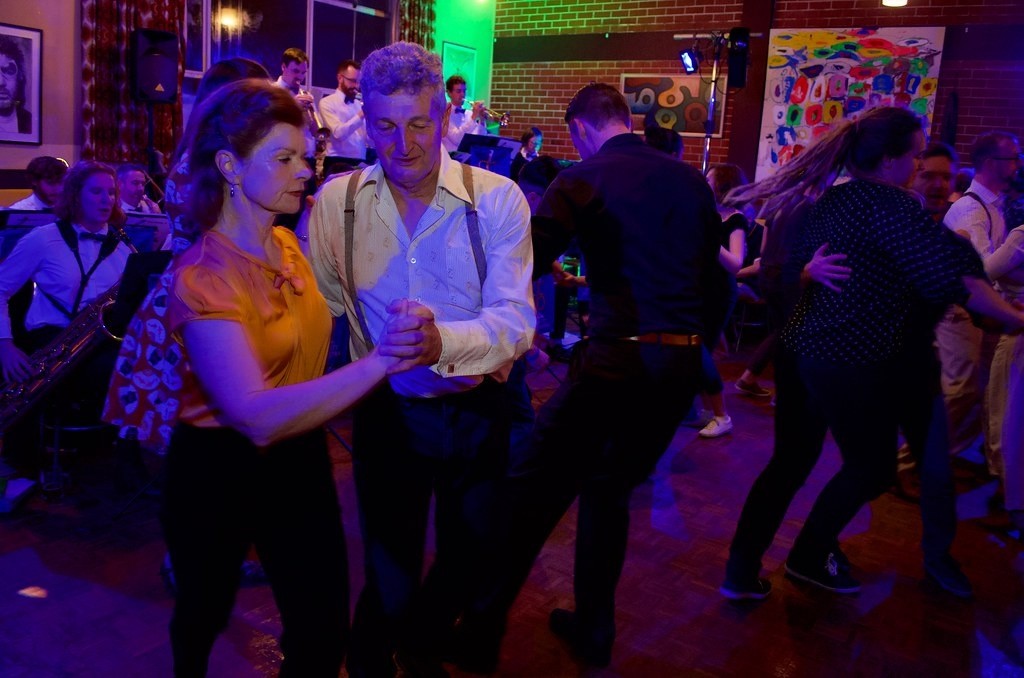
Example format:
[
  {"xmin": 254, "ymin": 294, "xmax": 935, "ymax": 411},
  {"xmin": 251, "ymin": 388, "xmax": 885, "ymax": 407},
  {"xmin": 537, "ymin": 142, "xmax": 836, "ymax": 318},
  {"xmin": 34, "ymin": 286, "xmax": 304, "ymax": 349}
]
[
  {"xmin": 0, "ymin": 46, "xmax": 688, "ymax": 506},
  {"xmin": 294, "ymin": 38, "xmax": 534, "ymax": 676},
  {"xmin": 448, "ymin": 85, "xmax": 721, "ymax": 674},
  {"xmin": 163, "ymin": 79, "xmax": 423, "ymax": 678},
  {"xmin": 690, "ymin": 161, "xmax": 752, "ymax": 437},
  {"xmin": 0, "ymin": 34, "xmax": 33, "ymax": 135},
  {"xmin": 721, "ymin": 109, "xmax": 1024, "ymax": 602}
]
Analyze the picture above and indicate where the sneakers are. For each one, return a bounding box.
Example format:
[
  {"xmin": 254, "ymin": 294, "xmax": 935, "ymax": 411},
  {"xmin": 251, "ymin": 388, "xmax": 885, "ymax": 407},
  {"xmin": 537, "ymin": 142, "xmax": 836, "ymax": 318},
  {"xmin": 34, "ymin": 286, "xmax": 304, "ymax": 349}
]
[
  {"xmin": 925, "ymin": 553, "xmax": 973, "ymax": 596},
  {"xmin": 699, "ymin": 416, "xmax": 733, "ymax": 438},
  {"xmin": 734, "ymin": 379, "xmax": 771, "ymax": 396},
  {"xmin": 691, "ymin": 409, "xmax": 714, "ymax": 428},
  {"xmin": 784, "ymin": 551, "xmax": 862, "ymax": 592},
  {"xmin": 719, "ymin": 576, "xmax": 772, "ymax": 599}
]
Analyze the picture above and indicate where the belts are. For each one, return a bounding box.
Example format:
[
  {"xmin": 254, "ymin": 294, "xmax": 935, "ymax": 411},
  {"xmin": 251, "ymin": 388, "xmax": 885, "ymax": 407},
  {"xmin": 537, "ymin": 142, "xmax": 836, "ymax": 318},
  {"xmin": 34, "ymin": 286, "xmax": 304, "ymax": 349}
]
[{"xmin": 625, "ymin": 335, "xmax": 700, "ymax": 344}]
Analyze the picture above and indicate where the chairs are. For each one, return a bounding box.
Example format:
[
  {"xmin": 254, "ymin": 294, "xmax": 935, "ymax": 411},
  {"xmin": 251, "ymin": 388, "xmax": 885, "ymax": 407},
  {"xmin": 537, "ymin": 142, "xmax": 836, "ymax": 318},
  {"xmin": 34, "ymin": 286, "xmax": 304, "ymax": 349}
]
[
  {"xmin": 38, "ymin": 415, "xmax": 109, "ymax": 487},
  {"xmin": 734, "ymin": 298, "xmax": 767, "ymax": 353}
]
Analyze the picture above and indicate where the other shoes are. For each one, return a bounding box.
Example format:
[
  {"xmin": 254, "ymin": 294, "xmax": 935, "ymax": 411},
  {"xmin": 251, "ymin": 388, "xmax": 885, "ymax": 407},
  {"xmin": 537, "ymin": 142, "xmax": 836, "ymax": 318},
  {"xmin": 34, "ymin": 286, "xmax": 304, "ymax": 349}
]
[
  {"xmin": 548, "ymin": 608, "xmax": 611, "ymax": 669},
  {"xmin": 898, "ymin": 479, "xmax": 921, "ymax": 504}
]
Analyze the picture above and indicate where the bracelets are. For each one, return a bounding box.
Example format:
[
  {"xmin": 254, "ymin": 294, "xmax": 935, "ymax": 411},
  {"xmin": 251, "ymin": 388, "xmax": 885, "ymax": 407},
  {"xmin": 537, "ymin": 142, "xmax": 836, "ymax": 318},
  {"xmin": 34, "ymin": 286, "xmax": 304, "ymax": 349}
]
[{"xmin": 295, "ymin": 230, "xmax": 307, "ymax": 243}]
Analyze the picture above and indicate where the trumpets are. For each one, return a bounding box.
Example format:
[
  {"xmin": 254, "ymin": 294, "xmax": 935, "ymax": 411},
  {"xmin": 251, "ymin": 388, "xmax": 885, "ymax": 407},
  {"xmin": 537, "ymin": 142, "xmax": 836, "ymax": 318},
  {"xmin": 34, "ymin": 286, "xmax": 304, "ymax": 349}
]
[
  {"xmin": 354, "ymin": 90, "xmax": 364, "ymax": 106},
  {"xmin": 293, "ymin": 78, "xmax": 332, "ymax": 141},
  {"xmin": 460, "ymin": 98, "xmax": 511, "ymax": 126}
]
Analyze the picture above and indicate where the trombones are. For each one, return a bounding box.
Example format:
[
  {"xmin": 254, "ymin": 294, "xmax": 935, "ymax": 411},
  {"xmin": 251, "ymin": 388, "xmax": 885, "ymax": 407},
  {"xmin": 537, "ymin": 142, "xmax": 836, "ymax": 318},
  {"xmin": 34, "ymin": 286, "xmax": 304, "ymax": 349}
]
[{"xmin": 138, "ymin": 169, "xmax": 165, "ymax": 209}]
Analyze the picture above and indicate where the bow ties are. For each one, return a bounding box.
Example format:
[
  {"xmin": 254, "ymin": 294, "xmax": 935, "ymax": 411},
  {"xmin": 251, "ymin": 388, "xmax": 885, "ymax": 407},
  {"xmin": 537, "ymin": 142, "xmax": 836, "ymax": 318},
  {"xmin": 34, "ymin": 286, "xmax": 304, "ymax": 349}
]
[
  {"xmin": 526, "ymin": 153, "xmax": 537, "ymax": 159},
  {"xmin": 129, "ymin": 208, "xmax": 142, "ymax": 213},
  {"xmin": 344, "ymin": 97, "xmax": 354, "ymax": 104},
  {"xmin": 455, "ymin": 108, "xmax": 465, "ymax": 114},
  {"xmin": 78, "ymin": 232, "xmax": 105, "ymax": 243},
  {"xmin": 286, "ymin": 86, "xmax": 299, "ymax": 94}
]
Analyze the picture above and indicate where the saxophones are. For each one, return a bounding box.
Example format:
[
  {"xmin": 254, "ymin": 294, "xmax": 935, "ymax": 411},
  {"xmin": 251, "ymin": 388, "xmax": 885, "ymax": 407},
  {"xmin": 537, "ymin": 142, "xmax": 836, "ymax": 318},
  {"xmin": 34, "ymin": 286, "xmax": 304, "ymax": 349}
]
[{"xmin": 0, "ymin": 220, "xmax": 140, "ymax": 431}]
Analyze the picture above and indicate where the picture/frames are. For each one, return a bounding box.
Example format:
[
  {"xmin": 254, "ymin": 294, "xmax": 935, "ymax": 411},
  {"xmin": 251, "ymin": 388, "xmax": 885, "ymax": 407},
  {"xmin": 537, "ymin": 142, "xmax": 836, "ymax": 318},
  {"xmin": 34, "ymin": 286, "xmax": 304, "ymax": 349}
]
[
  {"xmin": 441, "ymin": 41, "xmax": 477, "ymax": 102},
  {"xmin": 618, "ymin": 73, "xmax": 728, "ymax": 138},
  {"xmin": 0, "ymin": 21, "xmax": 45, "ymax": 146}
]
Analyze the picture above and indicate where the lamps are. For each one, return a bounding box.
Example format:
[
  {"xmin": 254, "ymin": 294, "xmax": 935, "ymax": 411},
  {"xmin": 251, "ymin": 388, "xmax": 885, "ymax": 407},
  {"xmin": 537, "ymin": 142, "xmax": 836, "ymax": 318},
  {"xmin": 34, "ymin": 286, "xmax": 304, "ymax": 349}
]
[{"xmin": 679, "ymin": 38, "xmax": 705, "ymax": 74}]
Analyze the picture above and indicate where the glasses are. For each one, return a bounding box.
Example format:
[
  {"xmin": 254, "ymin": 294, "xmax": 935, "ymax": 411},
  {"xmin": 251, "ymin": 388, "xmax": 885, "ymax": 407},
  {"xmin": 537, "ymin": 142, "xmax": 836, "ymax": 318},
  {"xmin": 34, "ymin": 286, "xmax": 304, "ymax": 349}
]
[
  {"xmin": 992, "ymin": 154, "xmax": 1021, "ymax": 162},
  {"xmin": 340, "ymin": 74, "xmax": 360, "ymax": 84}
]
